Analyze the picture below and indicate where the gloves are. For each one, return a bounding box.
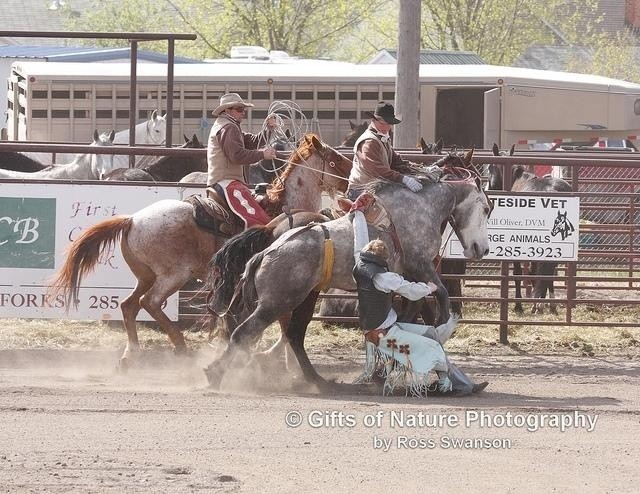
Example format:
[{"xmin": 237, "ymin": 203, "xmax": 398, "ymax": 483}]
[
  {"xmin": 264, "ymin": 148, "xmax": 276, "ymax": 160},
  {"xmin": 402, "ymin": 175, "xmax": 423, "ymax": 193},
  {"xmin": 268, "ymin": 118, "xmax": 277, "ymax": 130}
]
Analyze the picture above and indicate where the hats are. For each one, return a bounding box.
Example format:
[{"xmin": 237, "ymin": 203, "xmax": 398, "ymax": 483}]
[
  {"xmin": 367, "ymin": 100, "xmax": 402, "ymax": 125},
  {"xmin": 212, "ymin": 93, "xmax": 254, "ymax": 117}
]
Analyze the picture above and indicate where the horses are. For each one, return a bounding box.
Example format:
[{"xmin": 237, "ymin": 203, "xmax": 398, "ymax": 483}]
[
  {"xmin": 203, "ymin": 165, "xmax": 491, "ymax": 395},
  {"xmin": 112, "ymin": 109, "xmax": 167, "ymax": 171},
  {"xmin": 0, "ymin": 129, "xmax": 114, "ymax": 180},
  {"xmin": 340, "ymin": 120, "xmax": 371, "ymax": 147},
  {"xmin": 420, "ymin": 136, "xmax": 443, "ymax": 165},
  {"xmin": 179, "ymin": 144, "xmax": 493, "ymax": 385},
  {"xmin": 46, "ymin": 135, "xmax": 353, "ymax": 376},
  {"xmin": 99, "ymin": 134, "xmax": 208, "ymax": 182},
  {"xmin": 178, "ymin": 129, "xmax": 300, "ymax": 184},
  {"xmin": 487, "ymin": 142, "xmax": 572, "ymax": 316}
]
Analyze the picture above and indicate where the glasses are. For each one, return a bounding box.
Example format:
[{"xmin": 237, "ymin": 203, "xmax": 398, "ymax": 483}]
[{"xmin": 230, "ymin": 107, "xmax": 244, "ymax": 112}]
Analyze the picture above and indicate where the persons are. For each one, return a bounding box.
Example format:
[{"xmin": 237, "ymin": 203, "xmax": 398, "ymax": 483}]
[
  {"xmin": 349, "ymin": 202, "xmax": 490, "ymax": 398},
  {"xmin": 205, "ymin": 92, "xmax": 279, "ymax": 231},
  {"xmin": 343, "ymin": 100, "xmax": 441, "ymax": 201}
]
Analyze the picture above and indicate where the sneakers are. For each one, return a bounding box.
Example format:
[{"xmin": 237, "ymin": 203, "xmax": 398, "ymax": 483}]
[{"xmin": 448, "ymin": 381, "xmax": 489, "ymax": 396}]
